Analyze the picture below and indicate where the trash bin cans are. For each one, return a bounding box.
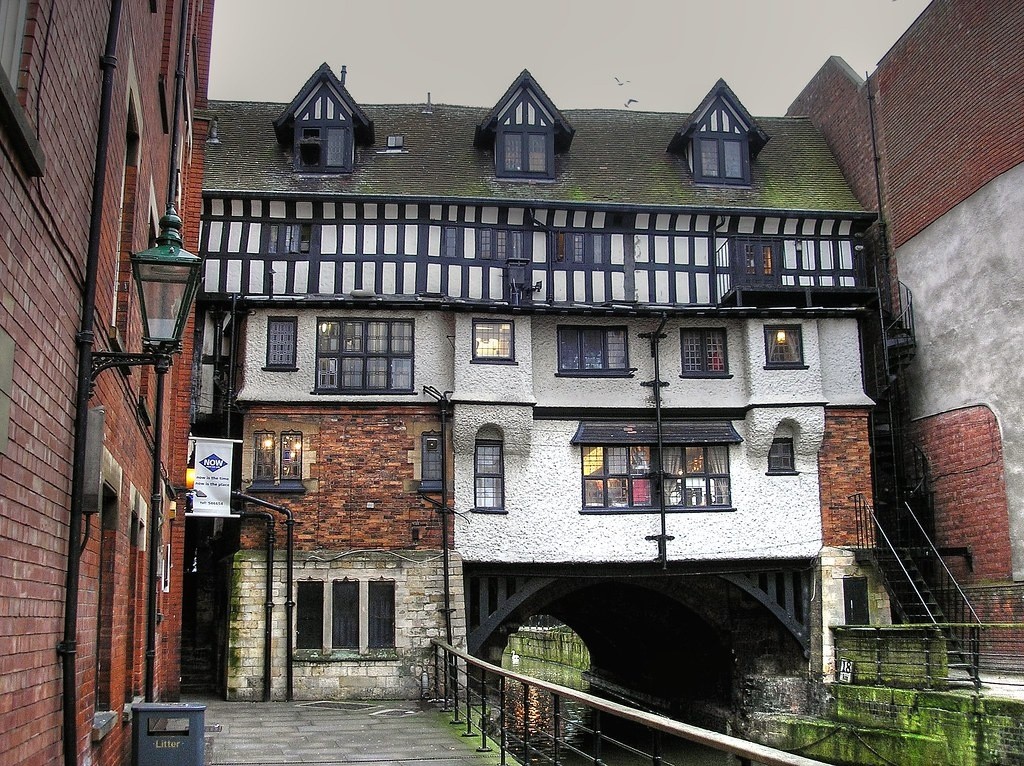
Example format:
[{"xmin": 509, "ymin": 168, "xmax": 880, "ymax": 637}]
[{"xmin": 130, "ymin": 703, "xmax": 208, "ymax": 766}]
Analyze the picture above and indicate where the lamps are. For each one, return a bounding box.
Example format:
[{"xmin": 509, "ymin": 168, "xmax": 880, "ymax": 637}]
[
  {"xmin": 185, "ymin": 441, "xmax": 196, "ymax": 489},
  {"xmin": 90, "ymin": 202, "xmax": 208, "ymax": 399},
  {"xmin": 194, "ymin": 115, "xmax": 221, "ymax": 147},
  {"xmin": 387, "ymin": 135, "xmax": 405, "ymax": 152}
]
